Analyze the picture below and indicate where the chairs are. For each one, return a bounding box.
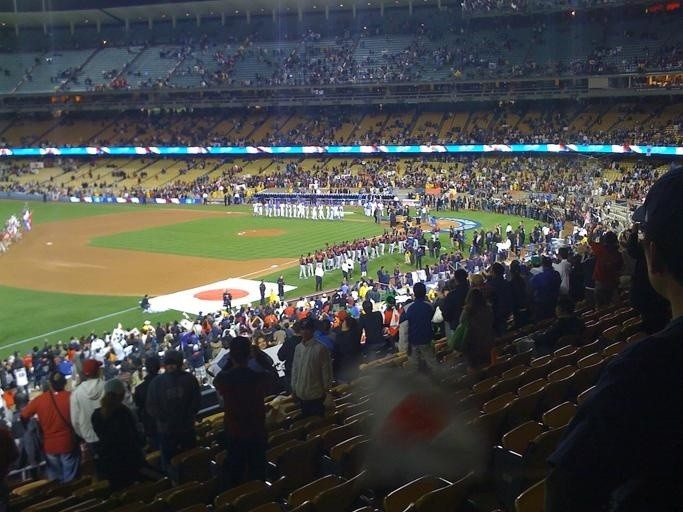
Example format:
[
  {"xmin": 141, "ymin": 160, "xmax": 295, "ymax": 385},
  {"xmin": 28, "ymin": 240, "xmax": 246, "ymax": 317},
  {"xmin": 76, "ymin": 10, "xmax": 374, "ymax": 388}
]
[
  {"xmin": 0, "ymin": 16, "xmax": 682, "ymax": 202},
  {"xmin": 570, "ymin": 202, "xmax": 644, "ymax": 235},
  {"xmin": 0, "ymin": 279, "xmax": 681, "ymax": 512}
]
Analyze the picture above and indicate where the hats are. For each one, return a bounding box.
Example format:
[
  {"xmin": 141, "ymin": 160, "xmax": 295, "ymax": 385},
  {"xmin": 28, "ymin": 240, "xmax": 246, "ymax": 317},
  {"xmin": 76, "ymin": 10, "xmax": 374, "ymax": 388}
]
[
  {"xmin": 105, "ymin": 378, "xmax": 125, "ymax": 396},
  {"xmin": 632, "ymin": 164, "xmax": 683, "ymax": 227},
  {"xmin": 82, "ymin": 358, "xmax": 102, "ymax": 375},
  {"xmin": 163, "ymin": 349, "xmax": 183, "ymax": 364},
  {"xmin": 49, "ymin": 371, "xmax": 65, "ymax": 382}
]
